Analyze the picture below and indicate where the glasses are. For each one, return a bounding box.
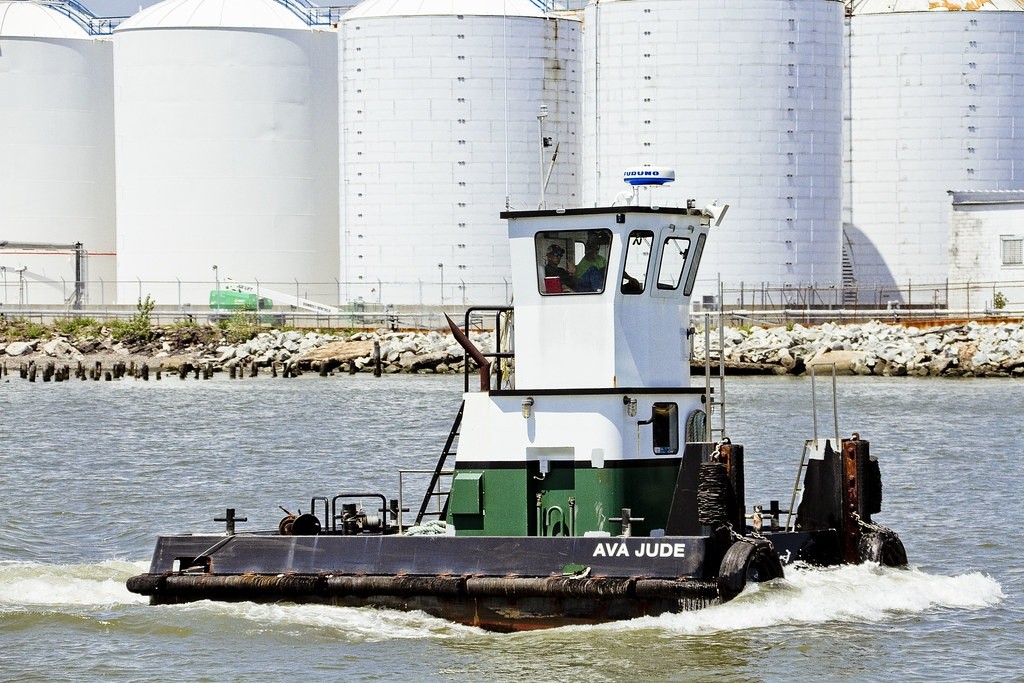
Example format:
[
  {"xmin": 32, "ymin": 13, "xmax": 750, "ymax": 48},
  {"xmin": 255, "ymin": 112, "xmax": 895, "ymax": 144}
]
[
  {"xmin": 585, "ymin": 245, "xmax": 595, "ymax": 250},
  {"xmin": 548, "ymin": 252, "xmax": 563, "ymax": 257}
]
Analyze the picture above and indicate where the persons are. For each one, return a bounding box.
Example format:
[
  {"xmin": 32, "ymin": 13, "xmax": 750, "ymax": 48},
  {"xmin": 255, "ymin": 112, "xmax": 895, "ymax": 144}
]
[
  {"xmin": 574, "ymin": 239, "xmax": 639, "ymax": 292},
  {"xmin": 543, "ymin": 244, "xmax": 577, "ymax": 293}
]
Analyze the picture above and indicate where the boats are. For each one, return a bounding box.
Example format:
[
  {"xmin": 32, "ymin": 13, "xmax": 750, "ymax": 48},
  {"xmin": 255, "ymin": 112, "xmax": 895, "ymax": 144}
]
[{"xmin": 124, "ymin": 160, "xmax": 910, "ymax": 631}]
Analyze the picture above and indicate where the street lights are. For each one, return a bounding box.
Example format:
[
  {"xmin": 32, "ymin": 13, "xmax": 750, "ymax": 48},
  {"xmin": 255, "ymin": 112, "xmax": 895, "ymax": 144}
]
[
  {"xmin": 212, "ymin": 264, "xmax": 222, "ymax": 318},
  {"xmin": 439, "ymin": 264, "xmax": 445, "ymax": 305}
]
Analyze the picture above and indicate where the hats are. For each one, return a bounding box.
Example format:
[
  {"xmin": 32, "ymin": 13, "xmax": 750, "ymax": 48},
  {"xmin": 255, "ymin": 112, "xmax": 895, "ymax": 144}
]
[{"xmin": 546, "ymin": 244, "xmax": 565, "ymax": 256}]
